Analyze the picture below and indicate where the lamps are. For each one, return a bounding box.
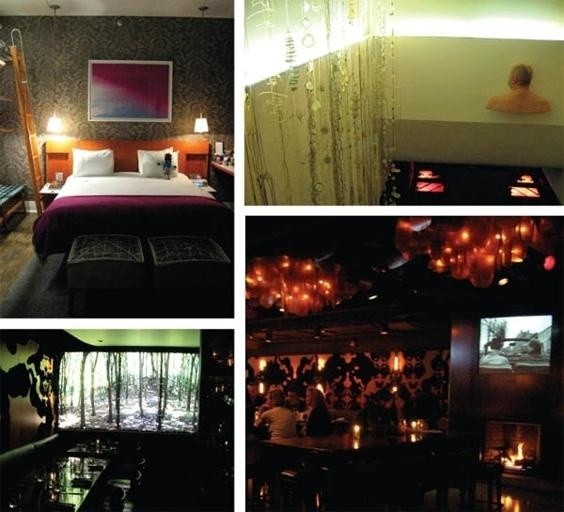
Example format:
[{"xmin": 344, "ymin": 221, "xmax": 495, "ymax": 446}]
[
  {"xmin": 245, "ymin": 221, "xmax": 358, "ymax": 321},
  {"xmin": 193, "ymin": 6, "xmax": 209, "ymax": 135},
  {"xmin": 45, "ymin": 3, "xmax": 63, "ymax": 134},
  {"xmin": 394, "ymin": 218, "xmax": 549, "ymax": 290}
]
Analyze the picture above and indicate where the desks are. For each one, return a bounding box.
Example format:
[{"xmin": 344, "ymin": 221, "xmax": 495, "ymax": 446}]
[{"xmin": 261, "ymin": 432, "xmax": 391, "ymax": 510}]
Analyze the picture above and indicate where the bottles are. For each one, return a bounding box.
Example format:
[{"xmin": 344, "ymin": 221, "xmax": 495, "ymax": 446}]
[
  {"xmin": 163, "ymin": 153, "xmax": 171, "ymax": 180},
  {"xmin": 78, "ymin": 438, "xmax": 114, "ymax": 452},
  {"xmin": 195, "ymin": 175, "xmax": 204, "ymax": 187},
  {"xmin": 45, "ymin": 455, "xmax": 94, "ymax": 487}
]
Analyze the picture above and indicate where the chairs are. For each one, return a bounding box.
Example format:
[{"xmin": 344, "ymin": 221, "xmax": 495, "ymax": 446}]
[{"xmin": 0, "ymin": 432, "xmax": 147, "ymax": 510}]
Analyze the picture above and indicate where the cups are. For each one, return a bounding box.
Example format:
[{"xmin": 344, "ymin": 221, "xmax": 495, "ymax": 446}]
[
  {"xmin": 397, "ymin": 217, "xmax": 554, "ymax": 288},
  {"xmin": 291, "ymin": 409, "xmax": 304, "ymax": 420},
  {"xmin": 399, "ymin": 418, "xmax": 425, "ymax": 429},
  {"xmin": 352, "ymin": 423, "xmax": 363, "ymax": 439},
  {"xmin": 352, "ymin": 440, "xmax": 361, "ymax": 451}
]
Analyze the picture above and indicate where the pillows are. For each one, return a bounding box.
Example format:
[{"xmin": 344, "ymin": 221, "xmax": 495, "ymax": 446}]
[{"xmin": 72, "ymin": 147, "xmax": 178, "ymax": 178}]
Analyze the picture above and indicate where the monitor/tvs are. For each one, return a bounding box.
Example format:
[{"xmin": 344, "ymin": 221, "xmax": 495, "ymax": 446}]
[{"xmin": 477, "ymin": 314, "xmax": 554, "ymax": 375}]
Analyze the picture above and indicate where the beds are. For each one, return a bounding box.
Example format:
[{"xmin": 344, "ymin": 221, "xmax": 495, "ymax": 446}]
[{"xmin": 34, "ymin": 141, "xmax": 229, "ymax": 249}]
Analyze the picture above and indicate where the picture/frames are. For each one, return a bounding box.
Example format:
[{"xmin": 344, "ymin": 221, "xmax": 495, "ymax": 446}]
[{"xmin": 86, "ymin": 59, "xmax": 173, "ymax": 124}]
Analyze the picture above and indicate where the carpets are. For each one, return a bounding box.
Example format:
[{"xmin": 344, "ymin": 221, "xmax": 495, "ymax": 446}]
[{"xmin": 0, "ymin": 252, "xmax": 178, "ymax": 317}]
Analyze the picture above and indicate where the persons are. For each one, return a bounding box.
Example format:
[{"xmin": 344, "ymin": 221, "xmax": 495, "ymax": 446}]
[
  {"xmin": 285, "ymin": 387, "xmax": 304, "ymax": 411},
  {"xmin": 254, "ymin": 389, "xmax": 299, "ymax": 471},
  {"xmin": 246, "ymin": 375, "xmax": 260, "ymax": 468},
  {"xmin": 525, "ymin": 334, "xmax": 546, "ymax": 356},
  {"xmin": 478, "ymin": 337, "xmax": 510, "ymax": 365},
  {"xmin": 486, "ymin": 63, "xmax": 551, "ymax": 113},
  {"xmin": 299, "ymin": 386, "xmax": 334, "ymax": 436},
  {"xmin": 351, "ymin": 387, "xmax": 399, "ymax": 428}
]
